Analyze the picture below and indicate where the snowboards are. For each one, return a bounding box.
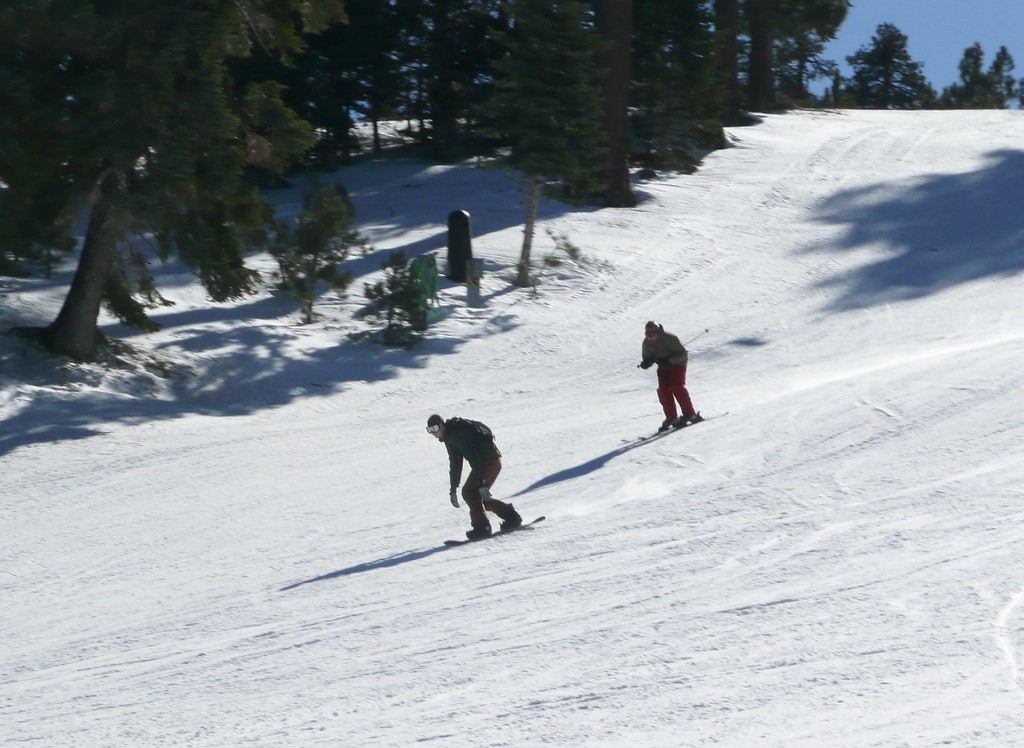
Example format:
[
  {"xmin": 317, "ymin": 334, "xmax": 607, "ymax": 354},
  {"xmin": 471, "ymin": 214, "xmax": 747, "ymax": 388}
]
[{"xmin": 444, "ymin": 516, "xmax": 545, "ymax": 546}]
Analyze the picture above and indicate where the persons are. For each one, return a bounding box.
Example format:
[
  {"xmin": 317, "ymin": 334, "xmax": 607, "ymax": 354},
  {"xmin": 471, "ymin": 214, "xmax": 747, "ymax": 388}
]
[
  {"xmin": 643, "ymin": 320, "xmax": 696, "ymax": 431},
  {"xmin": 427, "ymin": 414, "xmax": 522, "ymax": 541}
]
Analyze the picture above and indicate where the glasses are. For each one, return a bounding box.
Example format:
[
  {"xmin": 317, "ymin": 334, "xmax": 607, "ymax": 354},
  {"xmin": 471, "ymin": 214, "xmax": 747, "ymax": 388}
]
[
  {"xmin": 645, "ymin": 331, "xmax": 657, "ymax": 337},
  {"xmin": 426, "ymin": 424, "xmax": 440, "ymax": 434}
]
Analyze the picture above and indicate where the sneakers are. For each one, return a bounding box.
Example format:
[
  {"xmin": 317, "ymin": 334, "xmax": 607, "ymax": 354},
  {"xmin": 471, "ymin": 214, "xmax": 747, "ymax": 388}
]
[
  {"xmin": 500, "ymin": 503, "xmax": 522, "ymax": 529},
  {"xmin": 466, "ymin": 519, "xmax": 492, "ymax": 538}
]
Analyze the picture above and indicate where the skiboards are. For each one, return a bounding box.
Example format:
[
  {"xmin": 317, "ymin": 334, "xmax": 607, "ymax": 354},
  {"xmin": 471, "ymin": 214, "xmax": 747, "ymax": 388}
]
[{"xmin": 622, "ymin": 410, "xmax": 729, "ymax": 443}]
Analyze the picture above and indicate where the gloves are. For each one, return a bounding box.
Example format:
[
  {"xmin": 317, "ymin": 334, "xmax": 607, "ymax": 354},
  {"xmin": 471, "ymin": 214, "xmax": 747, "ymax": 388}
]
[
  {"xmin": 641, "ymin": 360, "xmax": 653, "ymax": 369},
  {"xmin": 478, "ymin": 485, "xmax": 492, "ymax": 502},
  {"xmin": 449, "ymin": 488, "xmax": 460, "ymax": 507}
]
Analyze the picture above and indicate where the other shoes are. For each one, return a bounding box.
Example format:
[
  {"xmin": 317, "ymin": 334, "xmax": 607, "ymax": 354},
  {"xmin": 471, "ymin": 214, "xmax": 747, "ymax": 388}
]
[
  {"xmin": 678, "ymin": 411, "xmax": 701, "ymax": 428},
  {"xmin": 662, "ymin": 416, "xmax": 678, "ymax": 428}
]
